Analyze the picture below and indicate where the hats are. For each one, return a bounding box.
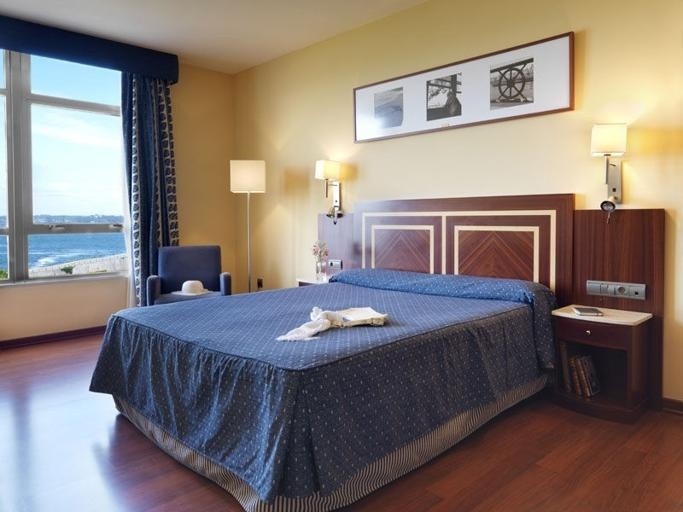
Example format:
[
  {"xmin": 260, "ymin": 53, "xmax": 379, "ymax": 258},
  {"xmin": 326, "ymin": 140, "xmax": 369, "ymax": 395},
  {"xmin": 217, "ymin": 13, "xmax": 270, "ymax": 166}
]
[{"xmin": 172, "ymin": 280, "xmax": 211, "ymax": 296}]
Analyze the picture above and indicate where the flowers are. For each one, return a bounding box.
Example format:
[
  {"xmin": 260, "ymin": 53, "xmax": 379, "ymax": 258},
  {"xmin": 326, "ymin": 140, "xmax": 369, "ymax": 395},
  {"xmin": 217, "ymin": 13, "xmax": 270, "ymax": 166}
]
[{"xmin": 310, "ymin": 238, "xmax": 329, "ymax": 273}]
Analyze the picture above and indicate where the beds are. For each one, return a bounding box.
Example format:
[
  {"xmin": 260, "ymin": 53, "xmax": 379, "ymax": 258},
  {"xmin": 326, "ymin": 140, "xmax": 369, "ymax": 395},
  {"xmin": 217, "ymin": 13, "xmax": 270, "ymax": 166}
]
[{"xmin": 106, "ymin": 192, "xmax": 576, "ymax": 512}]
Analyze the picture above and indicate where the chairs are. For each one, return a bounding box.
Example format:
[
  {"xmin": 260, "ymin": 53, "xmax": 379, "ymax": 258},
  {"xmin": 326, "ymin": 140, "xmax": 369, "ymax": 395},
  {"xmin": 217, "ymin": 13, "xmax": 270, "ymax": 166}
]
[{"xmin": 144, "ymin": 244, "xmax": 231, "ymax": 306}]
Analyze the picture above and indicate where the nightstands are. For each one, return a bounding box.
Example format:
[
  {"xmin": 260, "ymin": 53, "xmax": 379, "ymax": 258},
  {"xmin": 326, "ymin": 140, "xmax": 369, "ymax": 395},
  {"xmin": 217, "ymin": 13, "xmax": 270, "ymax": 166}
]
[{"xmin": 551, "ymin": 304, "xmax": 654, "ymax": 426}]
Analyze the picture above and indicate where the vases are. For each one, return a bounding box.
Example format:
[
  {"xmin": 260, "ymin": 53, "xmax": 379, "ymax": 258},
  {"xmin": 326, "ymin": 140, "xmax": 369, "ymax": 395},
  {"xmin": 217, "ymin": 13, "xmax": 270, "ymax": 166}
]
[{"xmin": 315, "ymin": 256, "xmax": 326, "ymax": 283}]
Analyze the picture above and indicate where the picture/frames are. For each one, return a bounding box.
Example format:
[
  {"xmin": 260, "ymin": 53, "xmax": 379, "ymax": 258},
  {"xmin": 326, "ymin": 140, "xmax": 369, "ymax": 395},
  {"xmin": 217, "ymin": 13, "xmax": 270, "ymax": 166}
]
[{"xmin": 351, "ymin": 31, "xmax": 574, "ymax": 145}]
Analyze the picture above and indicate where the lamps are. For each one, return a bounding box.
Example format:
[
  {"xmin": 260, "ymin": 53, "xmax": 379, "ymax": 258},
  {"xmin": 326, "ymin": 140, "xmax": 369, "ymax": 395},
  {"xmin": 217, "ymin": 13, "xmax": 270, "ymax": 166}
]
[
  {"xmin": 314, "ymin": 158, "xmax": 356, "ymax": 226},
  {"xmin": 228, "ymin": 158, "xmax": 266, "ymax": 292},
  {"xmin": 590, "ymin": 121, "xmax": 627, "ymax": 214}
]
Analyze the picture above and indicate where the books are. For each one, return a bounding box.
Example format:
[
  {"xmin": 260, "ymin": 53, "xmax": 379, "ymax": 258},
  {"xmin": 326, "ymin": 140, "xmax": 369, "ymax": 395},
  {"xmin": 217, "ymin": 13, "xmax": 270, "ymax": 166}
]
[{"xmin": 559, "ymin": 342, "xmax": 617, "ymax": 396}]
